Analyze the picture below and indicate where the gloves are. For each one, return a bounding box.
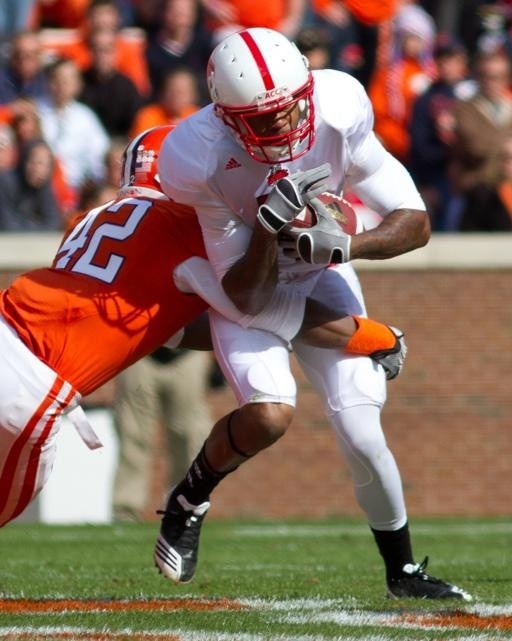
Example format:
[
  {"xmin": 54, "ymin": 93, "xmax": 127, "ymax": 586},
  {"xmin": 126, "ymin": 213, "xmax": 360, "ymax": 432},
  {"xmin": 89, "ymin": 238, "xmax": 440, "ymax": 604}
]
[
  {"xmin": 369, "ymin": 326, "xmax": 409, "ymax": 380},
  {"xmin": 256, "ymin": 161, "xmax": 352, "ymax": 266}
]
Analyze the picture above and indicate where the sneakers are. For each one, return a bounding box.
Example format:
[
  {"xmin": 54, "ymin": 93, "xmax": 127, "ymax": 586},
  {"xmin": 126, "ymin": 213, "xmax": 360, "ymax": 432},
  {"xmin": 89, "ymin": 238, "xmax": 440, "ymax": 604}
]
[
  {"xmin": 385, "ymin": 557, "xmax": 474, "ymax": 601},
  {"xmin": 153, "ymin": 480, "xmax": 210, "ymax": 585}
]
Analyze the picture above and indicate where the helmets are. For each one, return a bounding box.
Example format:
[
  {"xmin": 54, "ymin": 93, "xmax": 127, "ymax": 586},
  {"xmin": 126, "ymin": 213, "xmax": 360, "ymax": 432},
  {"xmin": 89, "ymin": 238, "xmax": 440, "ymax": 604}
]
[
  {"xmin": 205, "ymin": 27, "xmax": 316, "ymax": 164},
  {"xmin": 120, "ymin": 125, "xmax": 175, "ymax": 201}
]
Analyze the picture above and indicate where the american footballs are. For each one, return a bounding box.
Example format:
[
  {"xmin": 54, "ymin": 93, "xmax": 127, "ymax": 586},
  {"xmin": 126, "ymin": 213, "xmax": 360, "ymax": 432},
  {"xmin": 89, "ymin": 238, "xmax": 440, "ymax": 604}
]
[{"xmin": 258, "ymin": 192, "xmax": 357, "ymax": 239}]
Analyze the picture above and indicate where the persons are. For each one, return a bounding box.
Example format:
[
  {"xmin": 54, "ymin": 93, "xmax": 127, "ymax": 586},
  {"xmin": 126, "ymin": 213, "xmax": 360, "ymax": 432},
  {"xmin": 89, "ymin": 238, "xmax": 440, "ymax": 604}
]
[
  {"xmin": 194, "ymin": 1, "xmax": 511, "ymax": 232},
  {"xmin": 0, "ymin": 122, "xmax": 409, "ymax": 529},
  {"xmin": 156, "ymin": 27, "xmax": 474, "ymax": 612},
  {"xmin": 109, "ymin": 343, "xmax": 237, "ymax": 524},
  {"xmin": 0, "ymin": 0, "xmax": 218, "ymax": 233}
]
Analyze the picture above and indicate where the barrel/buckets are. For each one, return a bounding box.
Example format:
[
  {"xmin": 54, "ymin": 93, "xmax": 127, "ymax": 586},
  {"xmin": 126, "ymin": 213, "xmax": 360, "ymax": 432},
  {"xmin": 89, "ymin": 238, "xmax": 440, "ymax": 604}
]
[{"xmin": 33, "ymin": 397, "xmax": 122, "ymax": 529}]
